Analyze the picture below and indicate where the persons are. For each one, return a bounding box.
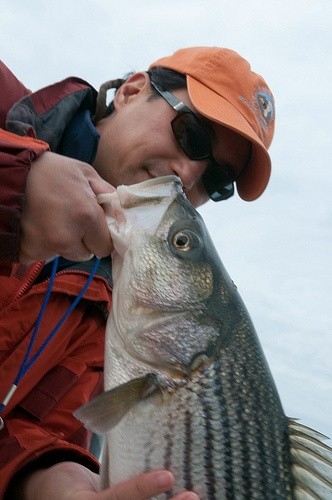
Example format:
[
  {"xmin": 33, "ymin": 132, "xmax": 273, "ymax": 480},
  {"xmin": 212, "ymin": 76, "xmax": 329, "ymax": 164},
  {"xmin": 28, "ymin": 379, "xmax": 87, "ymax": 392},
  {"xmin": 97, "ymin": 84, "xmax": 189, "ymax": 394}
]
[{"xmin": 0, "ymin": 46, "xmax": 277, "ymax": 500}]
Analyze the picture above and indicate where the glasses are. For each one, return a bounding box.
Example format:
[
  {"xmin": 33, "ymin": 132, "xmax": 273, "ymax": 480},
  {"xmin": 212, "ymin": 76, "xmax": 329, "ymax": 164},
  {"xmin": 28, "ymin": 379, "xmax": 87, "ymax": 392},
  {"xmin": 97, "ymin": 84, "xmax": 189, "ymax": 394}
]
[{"xmin": 151, "ymin": 79, "xmax": 235, "ymax": 203}]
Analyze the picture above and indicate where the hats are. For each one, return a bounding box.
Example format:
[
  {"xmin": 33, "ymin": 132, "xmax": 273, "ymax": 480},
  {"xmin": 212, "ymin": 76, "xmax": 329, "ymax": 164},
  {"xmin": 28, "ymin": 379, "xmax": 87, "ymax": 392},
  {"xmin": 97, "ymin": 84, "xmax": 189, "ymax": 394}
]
[{"xmin": 149, "ymin": 48, "xmax": 276, "ymax": 202}]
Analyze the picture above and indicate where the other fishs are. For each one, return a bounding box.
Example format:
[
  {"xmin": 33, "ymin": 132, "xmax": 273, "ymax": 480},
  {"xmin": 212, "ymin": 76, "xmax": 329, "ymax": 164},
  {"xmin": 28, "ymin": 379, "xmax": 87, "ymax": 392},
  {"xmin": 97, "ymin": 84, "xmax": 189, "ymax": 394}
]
[{"xmin": 72, "ymin": 174, "xmax": 332, "ymax": 499}]
